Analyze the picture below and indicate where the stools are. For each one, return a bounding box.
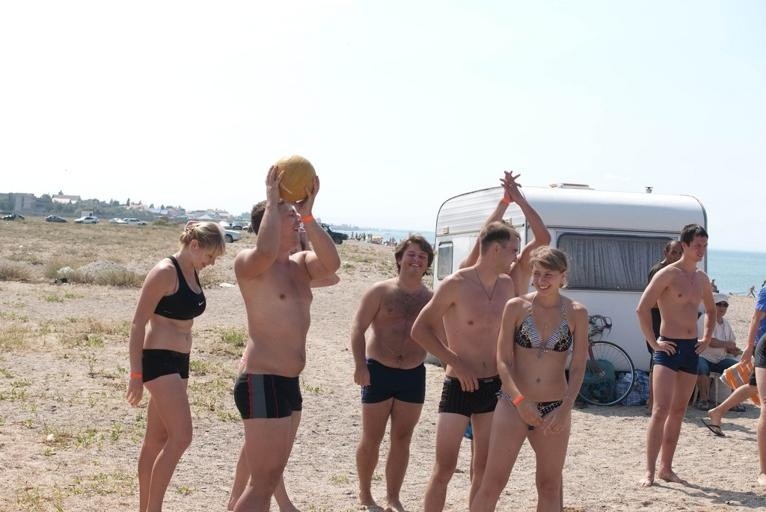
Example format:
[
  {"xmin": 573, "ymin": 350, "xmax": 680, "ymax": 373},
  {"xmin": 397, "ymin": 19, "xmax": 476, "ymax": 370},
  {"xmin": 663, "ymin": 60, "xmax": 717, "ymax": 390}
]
[{"xmin": 692, "ymin": 368, "xmax": 733, "ymax": 413}]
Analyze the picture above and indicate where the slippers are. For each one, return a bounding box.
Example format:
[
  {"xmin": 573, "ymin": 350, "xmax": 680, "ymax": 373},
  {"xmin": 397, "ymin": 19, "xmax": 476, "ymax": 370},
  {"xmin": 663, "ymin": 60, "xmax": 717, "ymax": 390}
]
[{"xmin": 701, "ymin": 416, "xmax": 725, "ymax": 437}]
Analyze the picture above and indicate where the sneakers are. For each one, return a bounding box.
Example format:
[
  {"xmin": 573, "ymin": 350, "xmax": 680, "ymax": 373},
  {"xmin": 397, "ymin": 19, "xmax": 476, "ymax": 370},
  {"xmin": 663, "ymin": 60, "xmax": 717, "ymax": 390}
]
[
  {"xmin": 696, "ymin": 398, "xmax": 710, "ymax": 410},
  {"xmin": 736, "ymin": 403, "xmax": 747, "ymax": 413}
]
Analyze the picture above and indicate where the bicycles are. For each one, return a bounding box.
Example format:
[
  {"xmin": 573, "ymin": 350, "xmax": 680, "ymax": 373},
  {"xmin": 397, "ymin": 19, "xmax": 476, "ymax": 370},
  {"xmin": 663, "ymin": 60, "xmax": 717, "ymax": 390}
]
[{"xmin": 568, "ymin": 314, "xmax": 639, "ymax": 408}]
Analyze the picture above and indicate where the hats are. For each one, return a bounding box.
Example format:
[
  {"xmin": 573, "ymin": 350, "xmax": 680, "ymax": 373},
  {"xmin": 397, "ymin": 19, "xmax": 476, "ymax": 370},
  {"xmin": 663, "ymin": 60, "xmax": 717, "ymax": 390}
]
[{"xmin": 713, "ymin": 292, "xmax": 729, "ymax": 305}]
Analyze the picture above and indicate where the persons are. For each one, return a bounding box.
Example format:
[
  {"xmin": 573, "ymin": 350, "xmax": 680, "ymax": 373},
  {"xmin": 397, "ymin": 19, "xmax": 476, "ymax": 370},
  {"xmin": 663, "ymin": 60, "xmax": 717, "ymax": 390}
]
[
  {"xmin": 459, "ymin": 170, "xmax": 552, "ymax": 481},
  {"xmin": 755, "ymin": 333, "xmax": 766, "ymax": 485},
  {"xmin": 412, "ymin": 221, "xmax": 521, "ymax": 512},
  {"xmin": 227, "ymin": 224, "xmax": 340, "ymax": 510},
  {"xmin": 468, "ymin": 245, "xmax": 590, "ymax": 512},
  {"xmin": 234, "ymin": 163, "xmax": 340, "ymax": 511},
  {"xmin": 749, "ymin": 284, "xmax": 757, "ymax": 295},
  {"xmin": 710, "ymin": 278, "xmax": 717, "ymax": 290},
  {"xmin": 648, "ymin": 240, "xmax": 683, "ymax": 413},
  {"xmin": 708, "ymin": 282, "xmax": 766, "ymax": 435},
  {"xmin": 124, "ymin": 221, "xmax": 225, "ymax": 511},
  {"xmin": 351, "ymin": 235, "xmax": 435, "ymax": 512},
  {"xmin": 350, "ymin": 232, "xmax": 400, "ymax": 245},
  {"xmin": 635, "ymin": 223, "xmax": 716, "ymax": 486},
  {"xmin": 695, "ymin": 293, "xmax": 748, "ymax": 413}
]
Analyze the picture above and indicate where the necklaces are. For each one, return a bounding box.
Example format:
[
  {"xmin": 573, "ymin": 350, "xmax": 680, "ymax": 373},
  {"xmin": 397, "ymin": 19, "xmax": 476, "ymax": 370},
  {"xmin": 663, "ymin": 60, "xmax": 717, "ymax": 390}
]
[
  {"xmin": 502, "ymin": 198, "xmax": 510, "ymax": 206},
  {"xmin": 475, "ymin": 266, "xmax": 500, "ymax": 301},
  {"xmin": 533, "ymin": 294, "xmax": 561, "ymax": 329},
  {"xmin": 176, "ymin": 253, "xmax": 198, "ymax": 294}
]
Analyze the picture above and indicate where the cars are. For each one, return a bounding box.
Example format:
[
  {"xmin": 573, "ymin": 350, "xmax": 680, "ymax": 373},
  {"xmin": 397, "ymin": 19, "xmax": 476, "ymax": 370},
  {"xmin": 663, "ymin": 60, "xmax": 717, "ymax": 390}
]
[
  {"xmin": 183, "ymin": 218, "xmax": 251, "ymax": 244},
  {"xmin": 0, "ymin": 211, "xmax": 24, "ymax": 222},
  {"xmin": 44, "ymin": 214, "xmax": 67, "ymax": 223},
  {"xmin": 108, "ymin": 216, "xmax": 146, "ymax": 226},
  {"xmin": 317, "ymin": 221, "xmax": 348, "ymax": 245},
  {"xmin": 72, "ymin": 214, "xmax": 99, "ymax": 225}
]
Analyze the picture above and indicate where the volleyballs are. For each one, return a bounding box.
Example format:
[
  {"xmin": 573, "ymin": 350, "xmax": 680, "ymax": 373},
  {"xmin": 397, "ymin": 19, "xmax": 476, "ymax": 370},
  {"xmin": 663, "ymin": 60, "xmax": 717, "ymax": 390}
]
[{"xmin": 272, "ymin": 156, "xmax": 317, "ymax": 203}]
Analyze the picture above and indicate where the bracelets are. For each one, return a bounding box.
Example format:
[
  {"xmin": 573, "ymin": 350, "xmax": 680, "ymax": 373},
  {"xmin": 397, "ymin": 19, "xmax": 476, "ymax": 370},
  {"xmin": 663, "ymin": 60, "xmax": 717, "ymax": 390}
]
[
  {"xmin": 512, "ymin": 393, "xmax": 524, "ymax": 406},
  {"xmin": 299, "ymin": 215, "xmax": 315, "ymax": 223},
  {"xmin": 563, "ymin": 395, "xmax": 577, "ymax": 401},
  {"xmin": 704, "ymin": 335, "xmax": 711, "ymax": 341},
  {"xmin": 129, "ymin": 373, "xmax": 144, "ymax": 378},
  {"xmin": 722, "ymin": 340, "xmax": 726, "ymax": 346},
  {"xmin": 748, "ymin": 342, "xmax": 754, "ymax": 345}
]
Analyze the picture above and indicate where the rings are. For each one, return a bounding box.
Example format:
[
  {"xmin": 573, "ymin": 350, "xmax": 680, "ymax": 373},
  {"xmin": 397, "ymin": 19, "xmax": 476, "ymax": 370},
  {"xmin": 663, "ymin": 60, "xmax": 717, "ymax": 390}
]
[
  {"xmin": 509, "ymin": 184, "xmax": 514, "ymax": 188},
  {"xmin": 555, "ymin": 426, "xmax": 559, "ymax": 430}
]
